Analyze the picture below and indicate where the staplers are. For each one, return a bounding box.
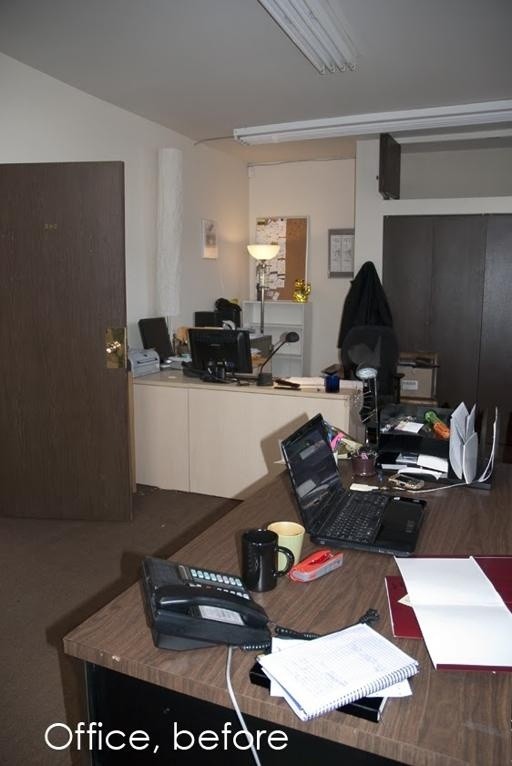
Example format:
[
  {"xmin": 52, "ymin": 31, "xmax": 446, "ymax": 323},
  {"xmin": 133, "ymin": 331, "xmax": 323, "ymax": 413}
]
[{"xmin": 290, "ymin": 549, "xmax": 344, "ymax": 582}]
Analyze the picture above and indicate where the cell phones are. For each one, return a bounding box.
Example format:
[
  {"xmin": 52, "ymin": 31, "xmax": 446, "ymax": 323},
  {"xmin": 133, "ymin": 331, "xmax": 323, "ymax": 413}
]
[{"xmin": 387, "ymin": 471, "xmax": 424, "ymax": 489}]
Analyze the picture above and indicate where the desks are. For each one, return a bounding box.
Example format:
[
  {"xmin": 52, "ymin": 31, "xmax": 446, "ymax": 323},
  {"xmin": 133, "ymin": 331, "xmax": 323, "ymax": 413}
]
[
  {"xmin": 64, "ymin": 455, "xmax": 512, "ymax": 766},
  {"xmin": 133, "ymin": 368, "xmax": 361, "ymax": 501}
]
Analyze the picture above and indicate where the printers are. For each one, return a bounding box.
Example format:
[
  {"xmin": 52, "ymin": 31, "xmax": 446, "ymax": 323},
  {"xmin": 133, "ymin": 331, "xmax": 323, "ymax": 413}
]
[{"xmin": 128, "ymin": 348, "xmax": 159, "ymax": 379}]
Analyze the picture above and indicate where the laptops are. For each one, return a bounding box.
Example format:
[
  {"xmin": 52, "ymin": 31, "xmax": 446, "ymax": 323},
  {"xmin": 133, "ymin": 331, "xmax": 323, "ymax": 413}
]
[{"xmin": 281, "ymin": 413, "xmax": 429, "ymax": 557}]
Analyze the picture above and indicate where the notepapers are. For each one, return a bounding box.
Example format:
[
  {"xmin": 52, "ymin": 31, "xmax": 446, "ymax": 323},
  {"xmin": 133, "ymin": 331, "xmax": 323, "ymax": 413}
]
[{"xmin": 255, "ymin": 218, "xmax": 287, "ymax": 301}]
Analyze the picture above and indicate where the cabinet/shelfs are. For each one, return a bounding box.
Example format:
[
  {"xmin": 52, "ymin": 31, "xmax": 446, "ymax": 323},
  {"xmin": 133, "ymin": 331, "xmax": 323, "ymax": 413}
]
[{"xmin": 240, "ymin": 298, "xmax": 312, "ymax": 379}]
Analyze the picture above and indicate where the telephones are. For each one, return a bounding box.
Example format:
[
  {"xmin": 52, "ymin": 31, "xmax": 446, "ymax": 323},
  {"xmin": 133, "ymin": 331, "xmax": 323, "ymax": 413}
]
[{"xmin": 139, "ymin": 554, "xmax": 381, "ymax": 652}]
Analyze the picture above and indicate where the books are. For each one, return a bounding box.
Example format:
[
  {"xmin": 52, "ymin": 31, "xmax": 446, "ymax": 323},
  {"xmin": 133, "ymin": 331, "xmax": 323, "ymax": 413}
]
[{"xmin": 249, "ymin": 622, "xmax": 420, "ymax": 725}]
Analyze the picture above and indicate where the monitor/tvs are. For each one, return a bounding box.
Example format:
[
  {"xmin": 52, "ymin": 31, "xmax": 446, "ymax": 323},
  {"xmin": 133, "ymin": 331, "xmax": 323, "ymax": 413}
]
[
  {"xmin": 188, "ymin": 328, "xmax": 253, "ymax": 384},
  {"xmin": 138, "ymin": 317, "xmax": 175, "ymax": 365}
]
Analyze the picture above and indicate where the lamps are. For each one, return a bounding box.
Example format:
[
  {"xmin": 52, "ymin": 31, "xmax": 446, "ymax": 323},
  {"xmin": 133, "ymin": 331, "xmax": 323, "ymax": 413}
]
[{"xmin": 248, "ymin": 244, "xmax": 281, "ymax": 267}]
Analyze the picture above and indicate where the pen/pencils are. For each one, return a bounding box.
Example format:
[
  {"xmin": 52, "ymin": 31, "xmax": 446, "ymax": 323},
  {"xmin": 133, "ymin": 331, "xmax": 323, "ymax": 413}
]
[
  {"xmin": 273, "ymin": 385, "xmax": 302, "ymax": 392},
  {"xmin": 324, "ymin": 427, "xmax": 343, "ymax": 452}
]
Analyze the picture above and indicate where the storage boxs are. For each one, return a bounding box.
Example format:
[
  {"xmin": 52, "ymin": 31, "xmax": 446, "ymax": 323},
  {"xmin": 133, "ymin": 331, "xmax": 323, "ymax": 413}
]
[{"xmin": 397, "ymin": 362, "xmax": 441, "ymax": 398}]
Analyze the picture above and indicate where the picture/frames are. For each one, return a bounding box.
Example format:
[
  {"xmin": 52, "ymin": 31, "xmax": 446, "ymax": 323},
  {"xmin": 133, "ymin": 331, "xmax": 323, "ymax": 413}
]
[{"xmin": 200, "ymin": 217, "xmax": 220, "ymax": 261}]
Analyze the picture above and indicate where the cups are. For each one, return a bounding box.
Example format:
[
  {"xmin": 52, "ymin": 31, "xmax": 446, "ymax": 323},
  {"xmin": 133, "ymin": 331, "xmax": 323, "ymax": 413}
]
[
  {"xmin": 241, "ymin": 530, "xmax": 295, "ymax": 591},
  {"xmin": 268, "ymin": 521, "xmax": 304, "ymax": 572}
]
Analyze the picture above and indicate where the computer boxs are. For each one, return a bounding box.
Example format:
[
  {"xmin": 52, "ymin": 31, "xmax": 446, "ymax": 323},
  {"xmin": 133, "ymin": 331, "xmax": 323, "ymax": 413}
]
[{"xmin": 196, "ymin": 312, "xmax": 240, "ymax": 328}]
